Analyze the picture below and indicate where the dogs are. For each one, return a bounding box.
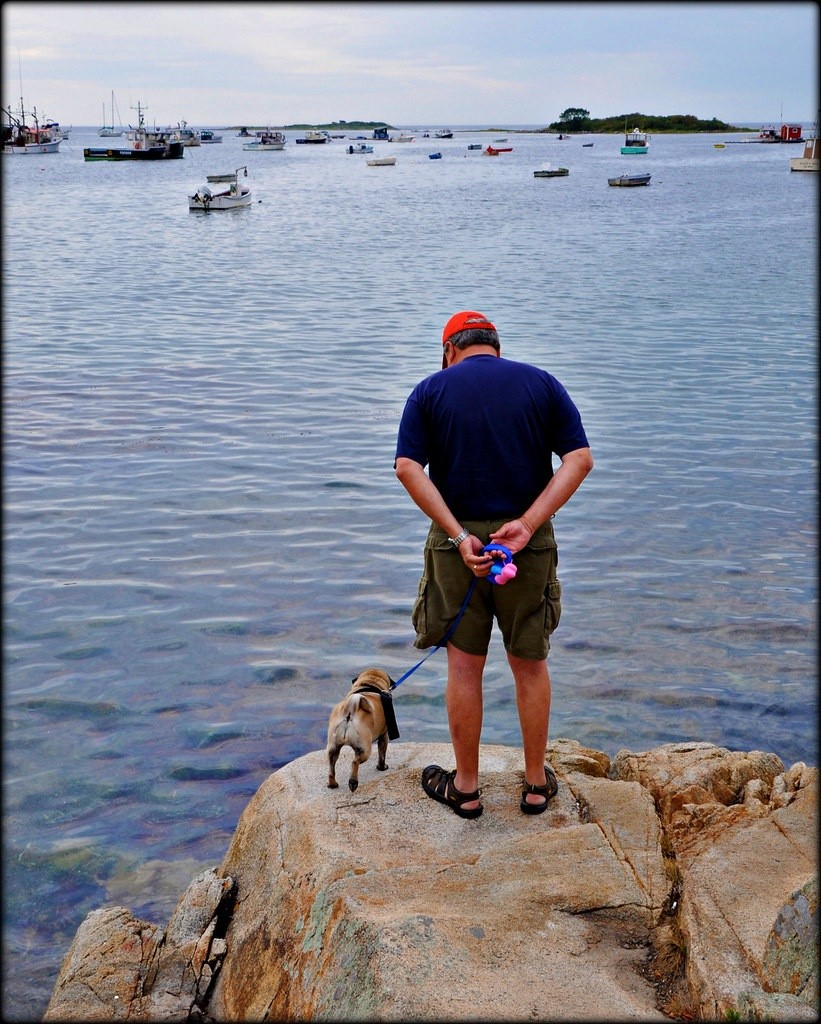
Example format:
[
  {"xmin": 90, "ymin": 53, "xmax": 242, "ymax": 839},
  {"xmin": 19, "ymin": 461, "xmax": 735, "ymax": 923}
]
[{"xmin": 326, "ymin": 668, "xmax": 398, "ymax": 793}]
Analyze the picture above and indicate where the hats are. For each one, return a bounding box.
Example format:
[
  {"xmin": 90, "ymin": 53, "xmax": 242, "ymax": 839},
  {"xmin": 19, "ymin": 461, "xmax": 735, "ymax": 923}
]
[{"xmin": 442, "ymin": 311, "xmax": 497, "ymax": 369}]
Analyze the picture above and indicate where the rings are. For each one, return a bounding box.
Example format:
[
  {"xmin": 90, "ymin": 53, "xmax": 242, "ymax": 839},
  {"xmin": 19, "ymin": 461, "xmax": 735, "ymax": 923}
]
[{"xmin": 473, "ymin": 564, "xmax": 477, "ymax": 571}]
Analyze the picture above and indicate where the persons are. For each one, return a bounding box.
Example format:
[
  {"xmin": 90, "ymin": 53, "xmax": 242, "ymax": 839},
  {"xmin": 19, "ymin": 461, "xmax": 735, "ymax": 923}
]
[
  {"xmin": 394, "ymin": 311, "xmax": 593, "ymax": 820},
  {"xmin": 157, "ymin": 135, "xmax": 165, "ymax": 144},
  {"xmin": 16, "ymin": 132, "xmax": 25, "ymax": 147}
]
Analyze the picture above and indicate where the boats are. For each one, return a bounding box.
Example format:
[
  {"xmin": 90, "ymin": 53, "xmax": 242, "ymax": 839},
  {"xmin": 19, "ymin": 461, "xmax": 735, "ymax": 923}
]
[
  {"xmin": 295, "ymin": 129, "xmax": 332, "ymax": 145},
  {"xmin": 345, "ymin": 143, "xmax": 373, "ymax": 154},
  {"xmin": 534, "ymin": 167, "xmax": 569, "ymax": 177},
  {"xmin": 243, "ymin": 128, "xmax": 288, "ymax": 152},
  {"xmin": 620, "ymin": 116, "xmax": 651, "ymax": 154},
  {"xmin": 188, "ymin": 166, "xmax": 253, "ymax": 211},
  {"xmin": 582, "ymin": 143, "xmax": 593, "ymax": 147},
  {"xmin": 714, "ymin": 143, "xmax": 725, "ymax": 148},
  {"xmin": 84, "ymin": 90, "xmax": 223, "ymax": 161},
  {"xmin": 429, "ymin": 152, "xmax": 442, "ymax": 159},
  {"xmin": 467, "ymin": 144, "xmax": 499, "ymax": 156},
  {"xmin": 206, "ymin": 174, "xmax": 238, "ymax": 183},
  {"xmin": 2, "ymin": 53, "xmax": 69, "ymax": 153},
  {"xmin": 366, "ymin": 157, "xmax": 397, "ymax": 166},
  {"xmin": 388, "ymin": 131, "xmax": 415, "ymax": 142},
  {"xmin": 422, "ymin": 128, "xmax": 453, "ymax": 139},
  {"xmin": 556, "ymin": 133, "xmax": 571, "ymax": 140},
  {"xmin": 329, "ymin": 134, "xmax": 346, "ymax": 139},
  {"xmin": 787, "ymin": 113, "xmax": 819, "ymax": 172},
  {"xmin": 607, "ymin": 173, "xmax": 651, "ymax": 186},
  {"xmin": 492, "ymin": 138, "xmax": 508, "ymax": 143}
]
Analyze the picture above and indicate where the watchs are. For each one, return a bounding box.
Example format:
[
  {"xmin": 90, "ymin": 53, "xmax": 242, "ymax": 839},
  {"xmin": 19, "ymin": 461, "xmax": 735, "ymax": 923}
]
[{"xmin": 447, "ymin": 527, "xmax": 469, "ymax": 550}]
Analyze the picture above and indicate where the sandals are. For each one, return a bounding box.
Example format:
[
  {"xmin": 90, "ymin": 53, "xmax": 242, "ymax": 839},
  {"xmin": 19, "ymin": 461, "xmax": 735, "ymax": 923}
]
[
  {"xmin": 521, "ymin": 765, "xmax": 558, "ymax": 814},
  {"xmin": 422, "ymin": 765, "xmax": 484, "ymax": 819}
]
